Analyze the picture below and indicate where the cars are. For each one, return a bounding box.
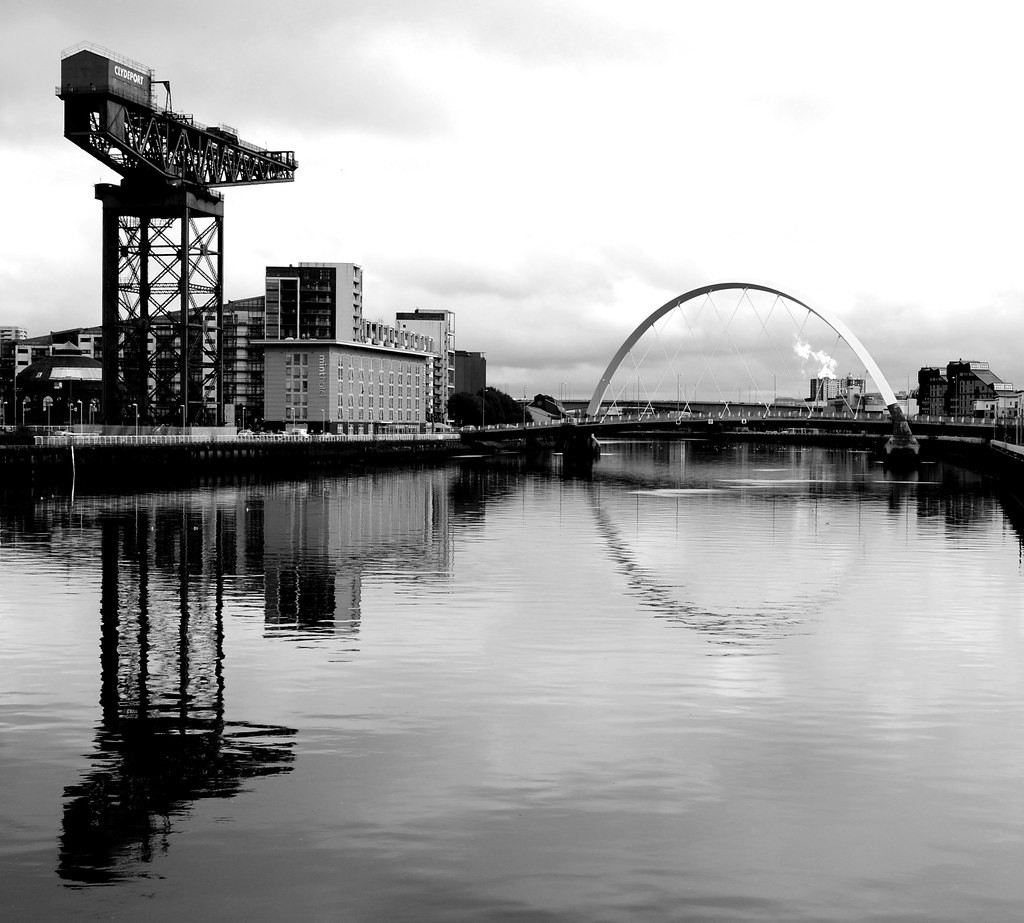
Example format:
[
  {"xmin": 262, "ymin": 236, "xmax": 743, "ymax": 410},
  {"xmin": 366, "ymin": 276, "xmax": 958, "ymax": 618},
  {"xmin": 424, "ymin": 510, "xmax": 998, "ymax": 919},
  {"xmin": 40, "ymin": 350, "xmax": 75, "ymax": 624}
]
[
  {"xmin": 238, "ymin": 428, "xmax": 346, "ymax": 439},
  {"xmin": 463, "ymin": 425, "xmax": 476, "ymax": 431}
]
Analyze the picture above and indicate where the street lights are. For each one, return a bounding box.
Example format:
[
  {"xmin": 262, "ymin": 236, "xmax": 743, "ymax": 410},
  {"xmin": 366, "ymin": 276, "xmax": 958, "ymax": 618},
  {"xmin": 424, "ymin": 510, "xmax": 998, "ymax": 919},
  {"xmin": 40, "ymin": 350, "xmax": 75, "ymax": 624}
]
[
  {"xmin": 637, "ymin": 374, "xmax": 644, "ymax": 419},
  {"xmin": 677, "ymin": 373, "xmax": 684, "ymax": 417},
  {"xmin": 523, "ymin": 385, "xmax": 529, "ymax": 427},
  {"xmin": 48, "ymin": 403, "xmax": 54, "ymax": 444},
  {"xmin": 560, "ymin": 381, "xmax": 567, "ymax": 422},
  {"xmin": 1, "ymin": 402, "xmax": 8, "ymax": 426},
  {"xmin": 76, "ymin": 401, "xmax": 82, "ymax": 445},
  {"xmin": 132, "ymin": 404, "xmax": 138, "ymax": 443},
  {"xmin": 22, "ymin": 401, "xmax": 26, "ymax": 427},
  {"xmin": 482, "ymin": 387, "xmax": 489, "ymax": 430},
  {"xmin": 321, "ymin": 409, "xmax": 325, "ymax": 435},
  {"xmin": 88, "ymin": 404, "xmax": 93, "ymax": 435},
  {"xmin": 770, "ymin": 374, "xmax": 776, "ymax": 413},
  {"xmin": 952, "ymin": 372, "xmax": 958, "ymax": 422},
  {"xmin": 180, "ymin": 404, "xmax": 186, "ymax": 443},
  {"xmin": 291, "ymin": 408, "xmax": 296, "ymax": 435},
  {"xmin": 242, "ymin": 407, "xmax": 245, "ymax": 431},
  {"xmin": 70, "ymin": 403, "xmax": 74, "ymax": 433}
]
